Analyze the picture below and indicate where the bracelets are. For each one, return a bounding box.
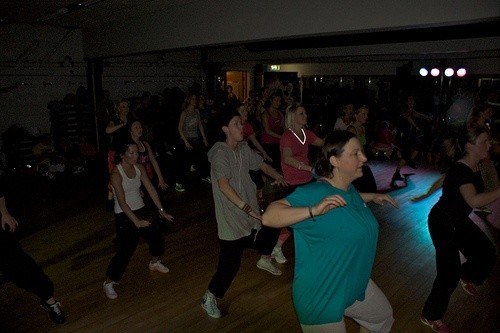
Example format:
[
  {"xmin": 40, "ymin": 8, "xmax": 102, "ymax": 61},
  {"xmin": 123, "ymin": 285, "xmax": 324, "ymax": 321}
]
[
  {"xmin": 158, "ymin": 208, "xmax": 165, "ymax": 216},
  {"xmin": 185, "ymin": 141, "xmax": 189, "ymax": 144},
  {"xmin": 242, "ymin": 203, "xmax": 247, "ymax": 210},
  {"xmin": 247, "ymin": 209, "xmax": 252, "ymax": 217},
  {"xmin": 123, "ymin": 122, "xmax": 125, "ymax": 127},
  {"xmin": 243, "ymin": 204, "xmax": 248, "ymax": 211},
  {"xmin": 245, "ymin": 207, "xmax": 250, "ymax": 213},
  {"xmin": 309, "ymin": 207, "xmax": 314, "ymax": 220}
]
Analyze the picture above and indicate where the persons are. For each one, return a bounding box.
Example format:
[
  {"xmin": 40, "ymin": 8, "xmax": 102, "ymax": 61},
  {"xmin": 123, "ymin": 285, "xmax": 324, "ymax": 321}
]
[{"xmin": 0, "ymin": 80, "xmax": 500, "ymax": 333}]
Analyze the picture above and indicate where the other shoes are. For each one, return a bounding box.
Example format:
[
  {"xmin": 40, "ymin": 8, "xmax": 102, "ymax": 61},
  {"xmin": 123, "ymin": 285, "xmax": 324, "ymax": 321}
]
[
  {"xmin": 201, "ymin": 176, "xmax": 211, "ymax": 182},
  {"xmin": 174, "ymin": 183, "xmax": 185, "ymax": 192}
]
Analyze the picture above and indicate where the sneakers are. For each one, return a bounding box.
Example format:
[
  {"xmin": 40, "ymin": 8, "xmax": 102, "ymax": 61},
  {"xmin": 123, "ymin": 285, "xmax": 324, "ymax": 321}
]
[
  {"xmin": 104, "ymin": 280, "xmax": 120, "ymax": 299},
  {"xmin": 40, "ymin": 299, "xmax": 65, "ymax": 324},
  {"xmin": 149, "ymin": 260, "xmax": 171, "ymax": 273},
  {"xmin": 420, "ymin": 317, "xmax": 449, "ymax": 333},
  {"xmin": 200, "ymin": 291, "xmax": 222, "ymax": 318},
  {"xmin": 271, "ymin": 247, "xmax": 288, "ymax": 264},
  {"xmin": 255, "ymin": 257, "xmax": 283, "ymax": 275},
  {"xmin": 459, "ymin": 279, "xmax": 481, "ymax": 296}
]
